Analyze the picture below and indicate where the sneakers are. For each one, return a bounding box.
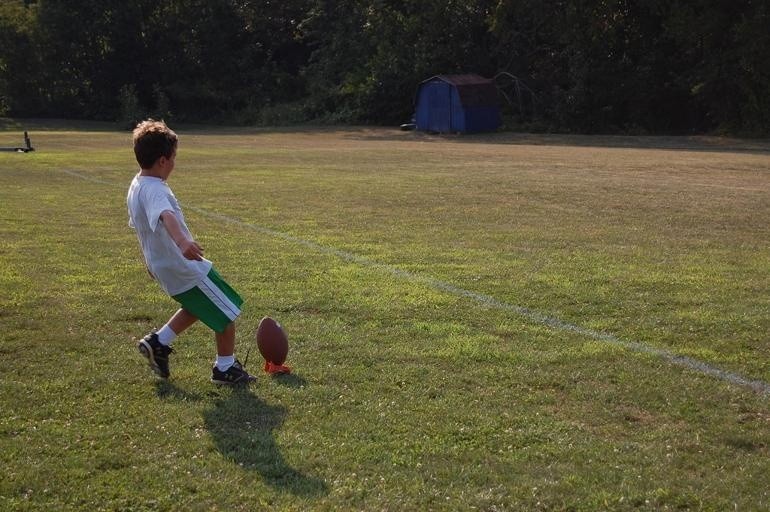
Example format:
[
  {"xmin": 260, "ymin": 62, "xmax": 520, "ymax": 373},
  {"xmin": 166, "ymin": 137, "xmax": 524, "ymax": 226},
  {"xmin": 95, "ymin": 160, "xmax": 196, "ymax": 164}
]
[
  {"xmin": 210, "ymin": 357, "xmax": 257, "ymax": 386},
  {"xmin": 138, "ymin": 332, "xmax": 177, "ymax": 378}
]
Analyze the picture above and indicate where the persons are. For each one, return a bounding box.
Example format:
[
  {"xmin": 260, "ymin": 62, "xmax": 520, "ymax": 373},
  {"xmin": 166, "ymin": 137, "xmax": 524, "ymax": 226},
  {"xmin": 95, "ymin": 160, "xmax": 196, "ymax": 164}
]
[{"xmin": 121, "ymin": 118, "xmax": 259, "ymax": 389}]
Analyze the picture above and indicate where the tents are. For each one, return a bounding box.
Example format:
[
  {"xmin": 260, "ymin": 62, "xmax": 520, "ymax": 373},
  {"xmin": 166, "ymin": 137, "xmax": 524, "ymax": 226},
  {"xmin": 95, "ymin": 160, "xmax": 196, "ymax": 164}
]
[{"xmin": 411, "ymin": 72, "xmax": 505, "ymax": 135}]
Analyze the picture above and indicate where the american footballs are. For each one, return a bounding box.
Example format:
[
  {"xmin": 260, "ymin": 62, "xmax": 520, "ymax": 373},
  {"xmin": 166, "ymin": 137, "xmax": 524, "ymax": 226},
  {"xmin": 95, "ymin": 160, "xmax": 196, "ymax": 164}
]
[{"xmin": 256, "ymin": 315, "xmax": 288, "ymax": 365}]
